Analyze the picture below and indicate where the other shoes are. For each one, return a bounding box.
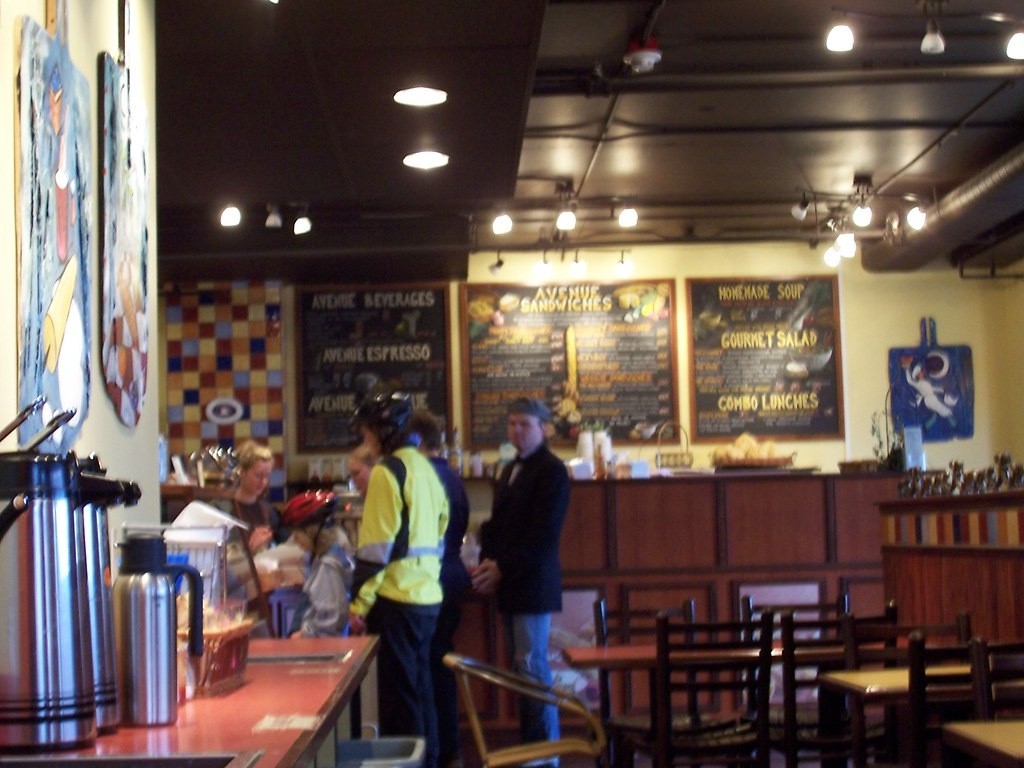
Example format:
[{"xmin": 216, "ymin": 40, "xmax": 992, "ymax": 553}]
[
  {"xmin": 517, "ymin": 754, "xmax": 559, "ymax": 768},
  {"xmin": 438, "ymin": 753, "xmax": 464, "ymax": 768}
]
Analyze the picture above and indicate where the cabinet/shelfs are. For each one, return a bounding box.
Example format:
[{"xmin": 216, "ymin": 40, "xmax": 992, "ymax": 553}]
[
  {"xmin": 42, "ymin": 635, "xmax": 383, "ymax": 767},
  {"xmin": 450, "ymin": 475, "xmax": 891, "ymax": 723}
]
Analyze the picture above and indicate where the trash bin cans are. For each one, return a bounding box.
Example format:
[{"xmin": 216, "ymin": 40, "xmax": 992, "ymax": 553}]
[{"xmin": 336, "ymin": 736, "xmax": 425, "ymax": 767}]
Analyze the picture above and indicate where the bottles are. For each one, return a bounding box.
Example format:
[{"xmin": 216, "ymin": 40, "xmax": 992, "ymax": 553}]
[
  {"xmin": 440, "ymin": 432, "xmax": 611, "ymax": 478},
  {"xmin": 897, "ymin": 453, "xmax": 1023, "ymax": 497}
]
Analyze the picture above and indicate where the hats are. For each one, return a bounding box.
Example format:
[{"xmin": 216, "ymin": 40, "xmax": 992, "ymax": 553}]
[{"xmin": 508, "ymin": 397, "xmax": 552, "ymax": 422}]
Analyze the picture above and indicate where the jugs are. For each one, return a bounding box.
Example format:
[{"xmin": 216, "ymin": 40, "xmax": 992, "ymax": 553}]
[
  {"xmin": 112, "ymin": 534, "xmax": 203, "ymax": 724},
  {"xmin": 1, "ymin": 450, "xmax": 141, "ymax": 752}
]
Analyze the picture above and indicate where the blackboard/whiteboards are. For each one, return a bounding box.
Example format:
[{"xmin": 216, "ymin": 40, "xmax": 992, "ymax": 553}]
[
  {"xmin": 291, "ymin": 284, "xmax": 454, "ymax": 456},
  {"xmin": 454, "ymin": 281, "xmax": 682, "ymax": 453},
  {"xmin": 684, "ymin": 273, "xmax": 845, "ymax": 445}
]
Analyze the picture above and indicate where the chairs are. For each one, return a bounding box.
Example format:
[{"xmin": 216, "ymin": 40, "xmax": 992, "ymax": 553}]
[{"xmin": 442, "ymin": 572, "xmax": 1024, "ymax": 768}]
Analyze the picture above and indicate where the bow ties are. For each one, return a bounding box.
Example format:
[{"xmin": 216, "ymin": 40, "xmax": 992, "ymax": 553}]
[{"xmin": 515, "ymin": 456, "xmax": 525, "ymax": 465}]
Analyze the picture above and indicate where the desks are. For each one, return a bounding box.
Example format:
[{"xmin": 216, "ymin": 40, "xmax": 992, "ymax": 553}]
[
  {"xmin": 939, "ymin": 720, "xmax": 1023, "ymax": 768},
  {"xmin": 562, "ymin": 637, "xmax": 972, "ymax": 768},
  {"xmin": 816, "ymin": 662, "xmax": 1024, "ymax": 768}
]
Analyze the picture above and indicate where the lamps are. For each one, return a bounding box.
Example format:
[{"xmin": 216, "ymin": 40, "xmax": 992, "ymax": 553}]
[
  {"xmin": 789, "ymin": 172, "xmax": 927, "ymax": 270},
  {"xmin": 492, "ymin": 177, "xmax": 640, "ymax": 232},
  {"xmin": 825, "ymin": 0, "xmax": 1024, "ymax": 60}
]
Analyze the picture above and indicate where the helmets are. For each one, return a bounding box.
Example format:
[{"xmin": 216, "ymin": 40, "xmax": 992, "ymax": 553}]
[
  {"xmin": 347, "ymin": 390, "xmax": 413, "ymax": 429},
  {"xmin": 279, "ymin": 489, "xmax": 338, "ymax": 528}
]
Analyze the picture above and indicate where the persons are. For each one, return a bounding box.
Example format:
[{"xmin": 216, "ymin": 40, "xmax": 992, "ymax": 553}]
[
  {"xmin": 213, "ymin": 439, "xmax": 291, "ymax": 562},
  {"xmin": 345, "ymin": 404, "xmax": 473, "ymax": 768},
  {"xmin": 278, "ymin": 490, "xmax": 355, "ymax": 638},
  {"xmin": 470, "ymin": 397, "xmax": 570, "ymax": 768}
]
